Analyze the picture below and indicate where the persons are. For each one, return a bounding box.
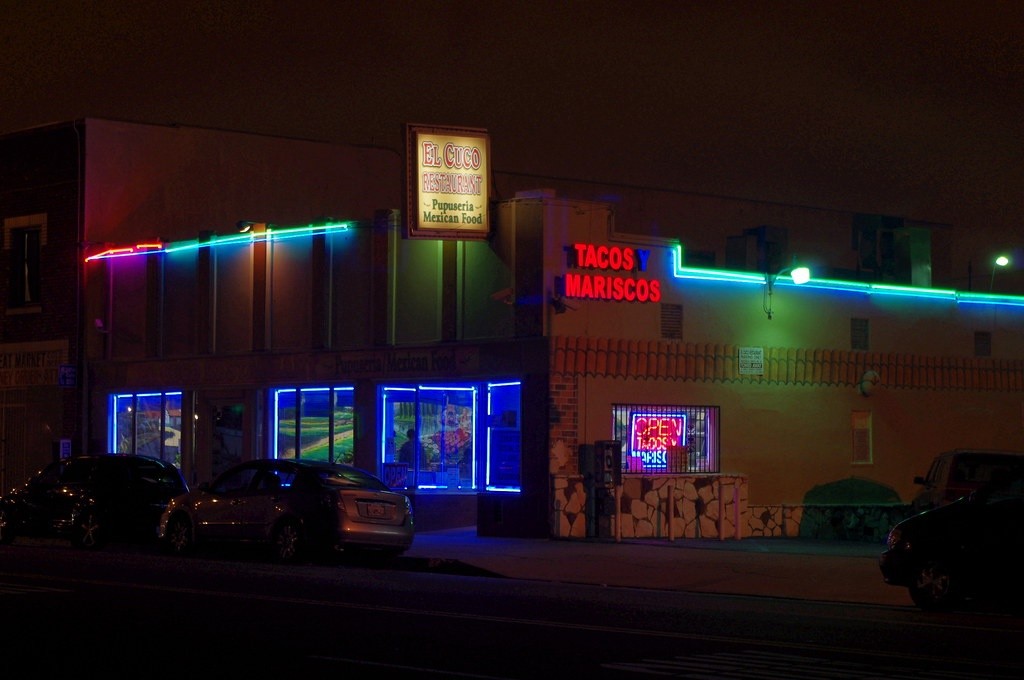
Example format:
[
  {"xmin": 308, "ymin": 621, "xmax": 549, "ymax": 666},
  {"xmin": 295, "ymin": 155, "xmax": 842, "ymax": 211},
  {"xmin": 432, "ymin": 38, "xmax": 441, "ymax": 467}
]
[
  {"xmin": 399, "ymin": 430, "xmax": 426, "ymax": 467},
  {"xmin": 458, "ymin": 450, "xmax": 472, "ymax": 479}
]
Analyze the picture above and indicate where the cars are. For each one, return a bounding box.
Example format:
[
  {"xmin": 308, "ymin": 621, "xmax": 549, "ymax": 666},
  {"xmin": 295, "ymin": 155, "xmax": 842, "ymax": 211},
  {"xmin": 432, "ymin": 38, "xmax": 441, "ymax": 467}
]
[
  {"xmin": 0, "ymin": 453, "xmax": 190, "ymax": 551},
  {"xmin": 880, "ymin": 457, "xmax": 1023, "ymax": 614},
  {"xmin": 158, "ymin": 459, "xmax": 415, "ymax": 565}
]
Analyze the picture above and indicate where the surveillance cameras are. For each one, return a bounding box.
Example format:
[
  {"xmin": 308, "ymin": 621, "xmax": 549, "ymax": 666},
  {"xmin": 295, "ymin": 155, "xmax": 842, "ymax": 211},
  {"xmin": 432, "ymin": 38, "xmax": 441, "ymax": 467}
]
[
  {"xmin": 94, "ymin": 319, "xmax": 103, "ymax": 330},
  {"xmin": 490, "ymin": 288, "xmax": 513, "ymax": 302},
  {"xmin": 560, "ymin": 296, "xmax": 582, "ymax": 312}
]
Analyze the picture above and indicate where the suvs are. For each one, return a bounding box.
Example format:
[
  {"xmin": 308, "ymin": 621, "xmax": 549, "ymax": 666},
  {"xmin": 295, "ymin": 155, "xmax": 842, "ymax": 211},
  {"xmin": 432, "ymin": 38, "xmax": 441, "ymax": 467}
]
[{"xmin": 912, "ymin": 448, "xmax": 1024, "ymax": 515}]
